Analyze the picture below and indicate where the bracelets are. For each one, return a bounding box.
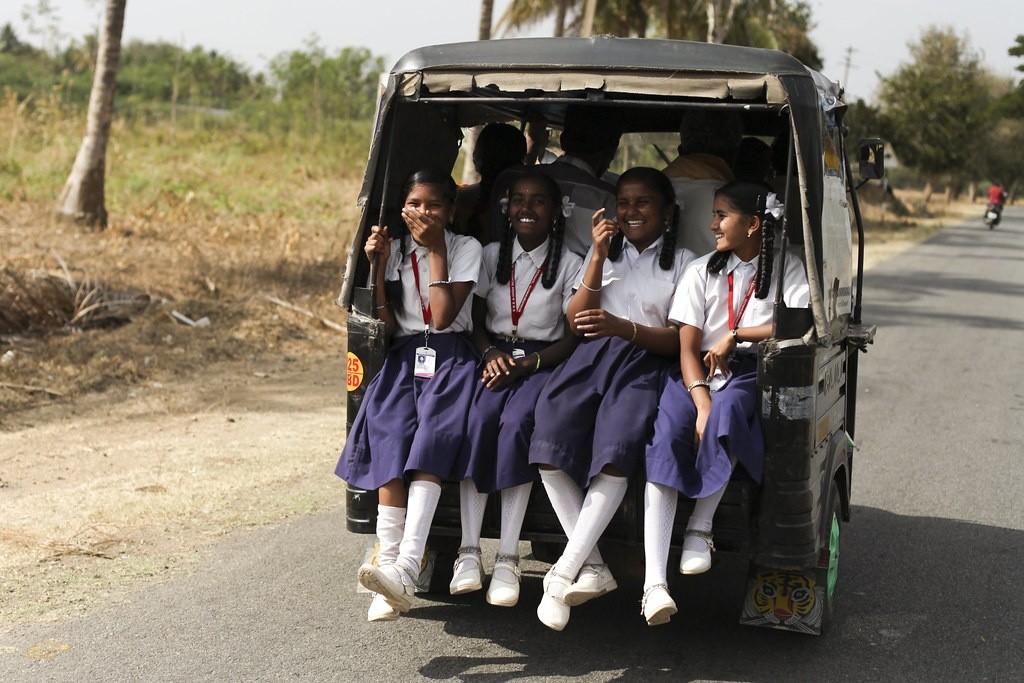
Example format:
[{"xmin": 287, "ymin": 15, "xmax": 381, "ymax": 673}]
[
  {"xmin": 687, "ymin": 380, "xmax": 709, "ymax": 392},
  {"xmin": 581, "ymin": 276, "xmax": 603, "ymax": 291},
  {"xmin": 481, "ymin": 345, "xmax": 497, "ymax": 360},
  {"xmin": 731, "ymin": 325, "xmax": 744, "ymax": 344},
  {"xmin": 374, "ymin": 301, "xmax": 389, "ymax": 309},
  {"xmin": 428, "ymin": 280, "xmax": 449, "ymax": 287},
  {"xmin": 531, "ymin": 351, "xmax": 541, "ymax": 373},
  {"xmin": 629, "ymin": 321, "xmax": 637, "ymax": 343}
]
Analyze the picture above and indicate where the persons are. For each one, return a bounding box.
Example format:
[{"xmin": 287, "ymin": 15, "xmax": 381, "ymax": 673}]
[
  {"xmin": 984, "ymin": 182, "xmax": 1005, "ymax": 225},
  {"xmin": 333, "ymin": 111, "xmax": 812, "ymax": 632}
]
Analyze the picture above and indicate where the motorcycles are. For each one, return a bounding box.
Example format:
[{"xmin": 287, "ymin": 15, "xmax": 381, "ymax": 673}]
[
  {"xmin": 340, "ymin": 36, "xmax": 884, "ymax": 635},
  {"xmin": 984, "ymin": 193, "xmax": 1008, "ymax": 228}
]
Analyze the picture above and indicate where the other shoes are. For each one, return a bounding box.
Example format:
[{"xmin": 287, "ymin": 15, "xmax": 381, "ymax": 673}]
[
  {"xmin": 449, "ymin": 554, "xmax": 486, "ymax": 594},
  {"xmin": 679, "ymin": 529, "xmax": 716, "ymax": 574},
  {"xmin": 537, "ymin": 563, "xmax": 570, "ymax": 631},
  {"xmin": 485, "ymin": 563, "xmax": 522, "ymax": 607},
  {"xmin": 565, "ymin": 565, "xmax": 617, "ymax": 606},
  {"xmin": 641, "ymin": 584, "xmax": 676, "ymax": 626},
  {"xmin": 357, "ymin": 562, "xmax": 418, "ymax": 611},
  {"xmin": 367, "ymin": 592, "xmax": 400, "ymax": 622}
]
